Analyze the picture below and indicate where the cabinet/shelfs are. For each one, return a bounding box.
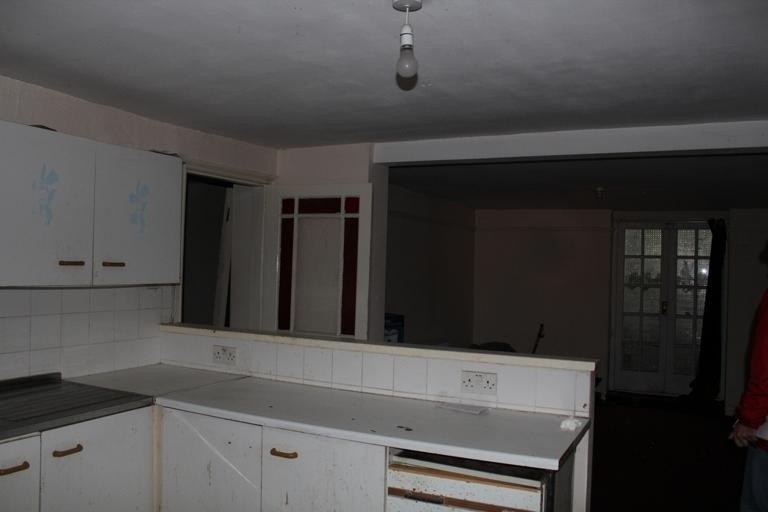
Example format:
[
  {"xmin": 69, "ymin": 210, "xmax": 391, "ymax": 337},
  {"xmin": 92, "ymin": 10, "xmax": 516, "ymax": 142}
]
[
  {"xmin": 386, "ymin": 449, "xmax": 573, "ymax": 512},
  {"xmin": 158, "ymin": 407, "xmax": 386, "ymax": 512},
  {"xmin": 0, "ymin": 405, "xmax": 156, "ymax": 512},
  {"xmin": 0, "ymin": 118, "xmax": 184, "ymax": 291}
]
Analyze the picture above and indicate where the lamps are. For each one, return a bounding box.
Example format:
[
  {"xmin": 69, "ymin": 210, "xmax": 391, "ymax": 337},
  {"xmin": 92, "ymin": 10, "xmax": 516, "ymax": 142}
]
[
  {"xmin": 392, "ymin": 0, "xmax": 423, "ymax": 79},
  {"xmin": 593, "ymin": 185, "xmax": 607, "ymax": 200}
]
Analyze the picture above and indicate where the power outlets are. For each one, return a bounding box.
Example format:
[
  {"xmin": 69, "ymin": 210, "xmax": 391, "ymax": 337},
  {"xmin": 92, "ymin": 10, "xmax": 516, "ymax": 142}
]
[
  {"xmin": 211, "ymin": 344, "xmax": 238, "ymax": 365},
  {"xmin": 460, "ymin": 371, "xmax": 498, "ymax": 396}
]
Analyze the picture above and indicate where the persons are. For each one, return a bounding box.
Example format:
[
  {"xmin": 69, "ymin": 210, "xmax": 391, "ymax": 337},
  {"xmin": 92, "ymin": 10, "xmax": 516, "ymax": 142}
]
[{"xmin": 727, "ymin": 246, "xmax": 768, "ymax": 511}]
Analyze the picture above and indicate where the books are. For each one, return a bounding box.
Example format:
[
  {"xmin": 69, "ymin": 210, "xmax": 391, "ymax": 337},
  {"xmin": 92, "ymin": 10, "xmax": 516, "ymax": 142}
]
[{"xmin": 732, "ymin": 417, "xmax": 768, "ymax": 438}]
